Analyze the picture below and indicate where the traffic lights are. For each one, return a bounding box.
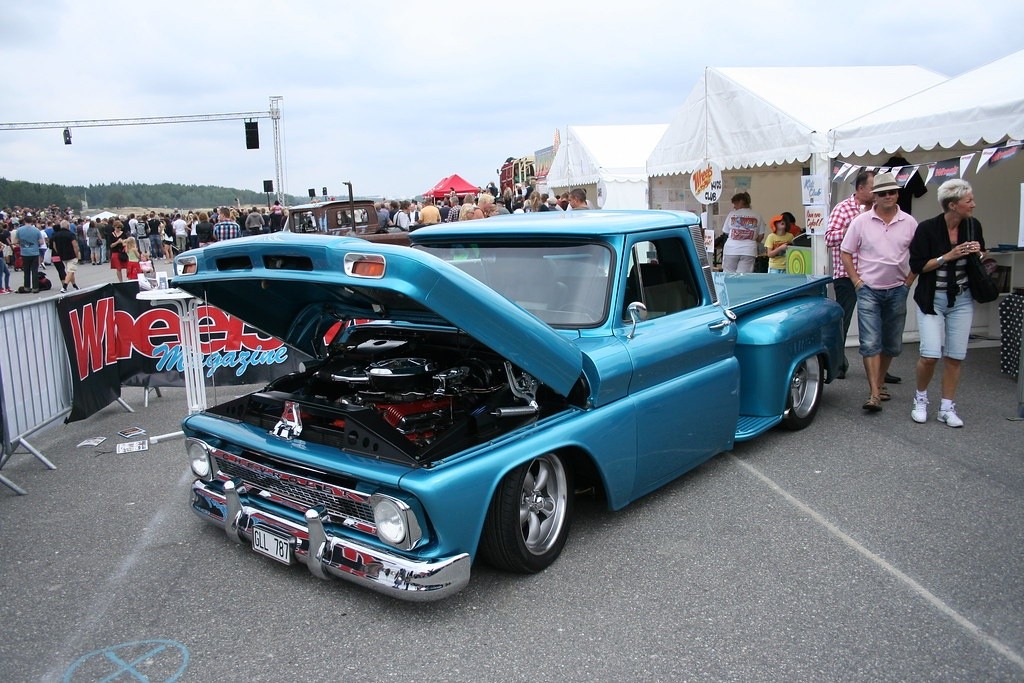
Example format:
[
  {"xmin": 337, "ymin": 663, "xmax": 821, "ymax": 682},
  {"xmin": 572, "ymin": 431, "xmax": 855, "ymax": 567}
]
[{"xmin": 63, "ymin": 129, "xmax": 73, "ymax": 145}]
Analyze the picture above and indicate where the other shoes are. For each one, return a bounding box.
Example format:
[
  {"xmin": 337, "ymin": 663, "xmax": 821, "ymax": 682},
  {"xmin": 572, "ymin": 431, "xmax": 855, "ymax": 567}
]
[
  {"xmin": 41, "ymin": 262, "xmax": 46, "ymax": 269},
  {"xmin": 74, "ymin": 287, "xmax": 81, "ymax": 291},
  {"xmin": 60, "ymin": 287, "xmax": 67, "ymax": 293},
  {"xmin": 31, "ymin": 289, "xmax": 39, "ymax": 293},
  {"xmin": 79, "ymin": 258, "xmax": 110, "ymax": 265},
  {"xmin": 884, "ymin": 372, "xmax": 901, "ymax": 384},
  {"xmin": 152, "ymin": 257, "xmax": 163, "ymax": 261},
  {"xmin": 24, "ymin": 288, "xmax": 31, "ymax": 293},
  {"xmin": 164, "ymin": 261, "xmax": 173, "ymax": 265}
]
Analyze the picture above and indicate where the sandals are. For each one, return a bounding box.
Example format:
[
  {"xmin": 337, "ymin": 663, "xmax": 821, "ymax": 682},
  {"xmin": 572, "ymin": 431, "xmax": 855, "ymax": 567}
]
[
  {"xmin": 862, "ymin": 394, "xmax": 882, "ymax": 412},
  {"xmin": 878, "ymin": 385, "xmax": 891, "ymax": 401}
]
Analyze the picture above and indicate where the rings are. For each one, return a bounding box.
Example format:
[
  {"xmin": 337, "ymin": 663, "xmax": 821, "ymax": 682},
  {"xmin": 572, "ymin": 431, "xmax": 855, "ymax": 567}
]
[{"xmin": 961, "ymin": 250, "xmax": 965, "ymax": 253}]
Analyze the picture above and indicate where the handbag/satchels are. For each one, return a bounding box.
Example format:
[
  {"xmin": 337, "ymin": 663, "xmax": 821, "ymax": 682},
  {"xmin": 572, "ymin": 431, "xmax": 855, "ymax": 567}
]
[
  {"xmin": 97, "ymin": 238, "xmax": 102, "ymax": 246},
  {"xmin": 118, "ymin": 251, "xmax": 128, "ymax": 262},
  {"xmin": 43, "ymin": 248, "xmax": 52, "ymax": 265},
  {"xmin": 964, "ymin": 217, "xmax": 1001, "ymax": 304}
]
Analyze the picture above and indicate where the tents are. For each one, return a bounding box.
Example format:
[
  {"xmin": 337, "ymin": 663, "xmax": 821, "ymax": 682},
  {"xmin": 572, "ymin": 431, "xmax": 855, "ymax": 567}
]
[
  {"xmin": 422, "ymin": 174, "xmax": 479, "ymax": 205},
  {"xmin": 645, "ymin": 66, "xmax": 952, "ymax": 273},
  {"xmin": 828, "ymin": 48, "xmax": 1024, "ymax": 346},
  {"xmin": 546, "ymin": 124, "xmax": 672, "ymax": 210}
]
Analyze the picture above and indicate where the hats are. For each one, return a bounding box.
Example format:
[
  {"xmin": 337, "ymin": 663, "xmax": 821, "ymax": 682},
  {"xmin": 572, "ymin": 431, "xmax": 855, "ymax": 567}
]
[
  {"xmin": 869, "ymin": 172, "xmax": 903, "ymax": 193},
  {"xmin": 78, "ymin": 219, "xmax": 84, "ymax": 223},
  {"xmin": 769, "ymin": 214, "xmax": 783, "ymax": 234},
  {"xmin": 547, "ymin": 196, "xmax": 558, "ymax": 205},
  {"xmin": 274, "ymin": 200, "xmax": 280, "ymax": 205},
  {"xmin": 522, "ymin": 199, "xmax": 532, "ymax": 209},
  {"xmin": 85, "ymin": 216, "xmax": 91, "ymax": 220}
]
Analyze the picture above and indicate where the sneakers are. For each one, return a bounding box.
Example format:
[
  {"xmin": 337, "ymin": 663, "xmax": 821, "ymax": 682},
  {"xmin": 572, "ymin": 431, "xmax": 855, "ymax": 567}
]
[
  {"xmin": 910, "ymin": 396, "xmax": 930, "ymax": 423},
  {"xmin": 5, "ymin": 287, "xmax": 14, "ymax": 293},
  {"xmin": 0, "ymin": 288, "xmax": 11, "ymax": 294},
  {"xmin": 936, "ymin": 403, "xmax": 964, "ymax": 427}
]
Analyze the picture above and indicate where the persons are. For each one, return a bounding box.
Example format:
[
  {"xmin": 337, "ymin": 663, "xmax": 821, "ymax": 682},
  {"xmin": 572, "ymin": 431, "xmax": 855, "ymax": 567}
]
[
  {"xmin": 722, "ymin": 191, "xmax": 765, "ymax": 273},
  {"xmin": 780, "ymin": 212, "xmax": 800, "ymax": 237},
  {"xmin": 909, "ymin": 179, "xmax": 986, "ymax": 427},
  {"xmin": 839, "ymin": 173, "xmax": 918, "ymax": 409},
  {"xmin": 824, "ymin": 171, "xmax": 903, "ymax": 383},
  {"xmin": 764, "ymin": 214, "xmax": 795, "ymax": 273},
  {"xmin": 362, "ymin": 182, "xmax": 594, "ymax": 259},
  {"xmin": 0, "ymin": 197, "xmax": 352, "ymax": 291}
]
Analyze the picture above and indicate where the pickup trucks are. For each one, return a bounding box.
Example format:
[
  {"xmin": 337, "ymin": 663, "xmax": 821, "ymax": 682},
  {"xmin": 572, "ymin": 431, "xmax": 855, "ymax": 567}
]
[{"xmin": 168, "ymin": 206, "xmax": 848, "ymax": 603}]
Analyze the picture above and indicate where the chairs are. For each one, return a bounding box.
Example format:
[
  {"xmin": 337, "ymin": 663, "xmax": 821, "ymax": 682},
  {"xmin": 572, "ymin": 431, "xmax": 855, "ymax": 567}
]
[
  {"xmin": 498, "ymin": 259, "xmax": 566, "ymax": 308},
  {"xmin": 624, "ymin": 263, "xmax": 688, "ymax": 317}
]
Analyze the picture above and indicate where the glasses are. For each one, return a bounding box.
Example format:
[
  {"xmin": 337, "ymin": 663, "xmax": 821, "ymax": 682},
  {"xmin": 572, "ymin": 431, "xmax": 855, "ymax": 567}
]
[
  {"xmin": 874, "ymin": 189, "xmax": 898, "ymax": 197},
  {"xmin": 116, "ymin": 227, "xmax": 121, "ymax": 228}
]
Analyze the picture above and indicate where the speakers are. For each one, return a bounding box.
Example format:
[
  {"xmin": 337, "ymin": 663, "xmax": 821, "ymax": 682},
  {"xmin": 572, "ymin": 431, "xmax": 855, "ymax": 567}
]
[
  {"xmin": 63, "ymin": 130, "xmax": 71, "ymax": 144},
  {"xmin": 308, "ymin": 189, "xmax": 315, "ymax": 197},
  {"xmin": 323, "ymin": 187, "xmax": 327, "ymax": 195},
  {"xmin": 264, "ymin": 180, "xmax": 273, "ymax": 192},
  {"xmin": 245, "ymin": 122, "xmax": 259, "ymax": 150}
]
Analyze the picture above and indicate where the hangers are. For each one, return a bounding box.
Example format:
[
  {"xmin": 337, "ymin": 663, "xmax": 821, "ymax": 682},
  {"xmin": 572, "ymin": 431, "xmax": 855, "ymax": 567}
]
[{"xmin": 894, "ymin": 150, "xmax": 904, "ymax": 157}]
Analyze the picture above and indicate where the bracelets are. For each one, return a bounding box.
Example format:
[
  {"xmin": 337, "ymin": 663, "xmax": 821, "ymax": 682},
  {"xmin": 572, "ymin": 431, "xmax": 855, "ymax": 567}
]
[
  {"xmin": 937, "ymin": 256, "xmax": 944, "ymax": 265},
  {"xmin": 854, "ymin": 280, "xmax": 863, "ymax": 288}
]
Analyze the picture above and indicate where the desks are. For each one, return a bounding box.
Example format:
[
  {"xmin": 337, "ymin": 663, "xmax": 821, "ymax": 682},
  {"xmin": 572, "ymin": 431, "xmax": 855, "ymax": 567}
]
[
  {"xmin": 998, "ymin": 293, "xmax": 1024, "ymax": 380},
  {"xmin": 137, "ymin": 290, "xmax": 209, "ymax": 444}
]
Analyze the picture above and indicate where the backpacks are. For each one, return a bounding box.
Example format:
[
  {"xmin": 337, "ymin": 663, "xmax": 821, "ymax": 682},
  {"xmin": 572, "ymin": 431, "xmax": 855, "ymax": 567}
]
[{"xmin": 37, "ymin": 271, "xmax": 52, "ymax": 290}]
